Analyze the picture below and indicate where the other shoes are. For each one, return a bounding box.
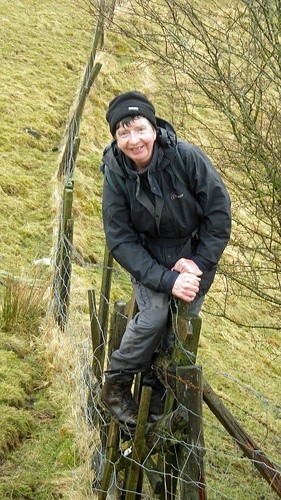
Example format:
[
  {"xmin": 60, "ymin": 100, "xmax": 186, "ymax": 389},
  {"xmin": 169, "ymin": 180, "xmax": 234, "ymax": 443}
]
[{"xmin": 100, "ymin": 379, "xmax": 139, "ymax": 429}]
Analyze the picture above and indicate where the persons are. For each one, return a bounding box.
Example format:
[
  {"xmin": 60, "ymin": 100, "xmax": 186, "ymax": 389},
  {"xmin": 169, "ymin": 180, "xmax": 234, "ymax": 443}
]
[{"xmin": 99, "ymin": 92, "xmax": 232, "ymax": 435}]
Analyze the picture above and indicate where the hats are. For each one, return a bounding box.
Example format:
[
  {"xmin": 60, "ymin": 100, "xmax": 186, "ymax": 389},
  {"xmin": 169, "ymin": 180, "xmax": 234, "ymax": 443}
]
[{"xmin": 106, "ymin": 90, "xmax": 158, "ymax": 134}]
